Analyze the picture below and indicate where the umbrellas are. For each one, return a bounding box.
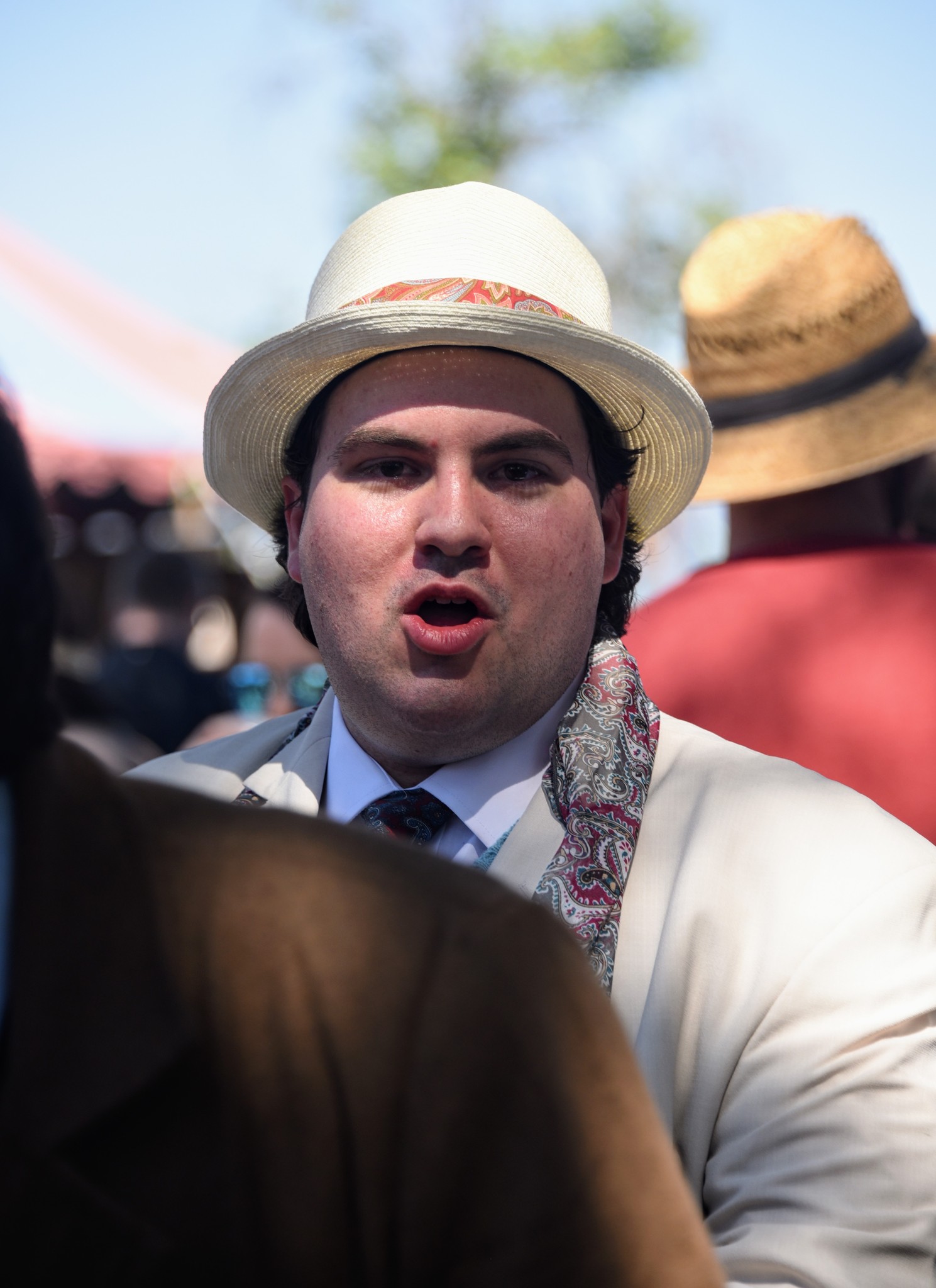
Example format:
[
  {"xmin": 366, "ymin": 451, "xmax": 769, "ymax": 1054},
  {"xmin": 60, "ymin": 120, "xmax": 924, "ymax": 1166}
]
[{"xmin": 1, "ymin": 226, "xmax": 254, "ymax": 503}]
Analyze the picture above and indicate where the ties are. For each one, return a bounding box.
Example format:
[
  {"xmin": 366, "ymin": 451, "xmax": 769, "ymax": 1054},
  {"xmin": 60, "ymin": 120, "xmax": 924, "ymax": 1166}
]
[{"xmin": 361, "ymin": 789, "xmax": 450, "ymax": 852}]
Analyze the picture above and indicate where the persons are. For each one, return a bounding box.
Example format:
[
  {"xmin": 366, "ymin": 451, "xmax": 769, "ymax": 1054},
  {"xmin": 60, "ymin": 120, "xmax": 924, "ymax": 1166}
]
[
  {"xmin": 612, "ymin": 208, "xmax": 936, "ymax": 854},
  {"xmin": 0, "ymin": 402, "xmax": 730, "ymax": 1287},
  {"xmin": 123, "ymin": 182, "xmax": 936, "ymax": 1288}
]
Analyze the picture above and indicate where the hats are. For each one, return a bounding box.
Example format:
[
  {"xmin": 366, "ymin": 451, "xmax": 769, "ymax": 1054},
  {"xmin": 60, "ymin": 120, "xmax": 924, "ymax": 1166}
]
[
  {"xmin": 673, "ymin": 209, "xmax": 936, "ymax": 502},
  {"xmin": 203, "ymin": 182, "xmax": 711, "ymax": 544}
]
